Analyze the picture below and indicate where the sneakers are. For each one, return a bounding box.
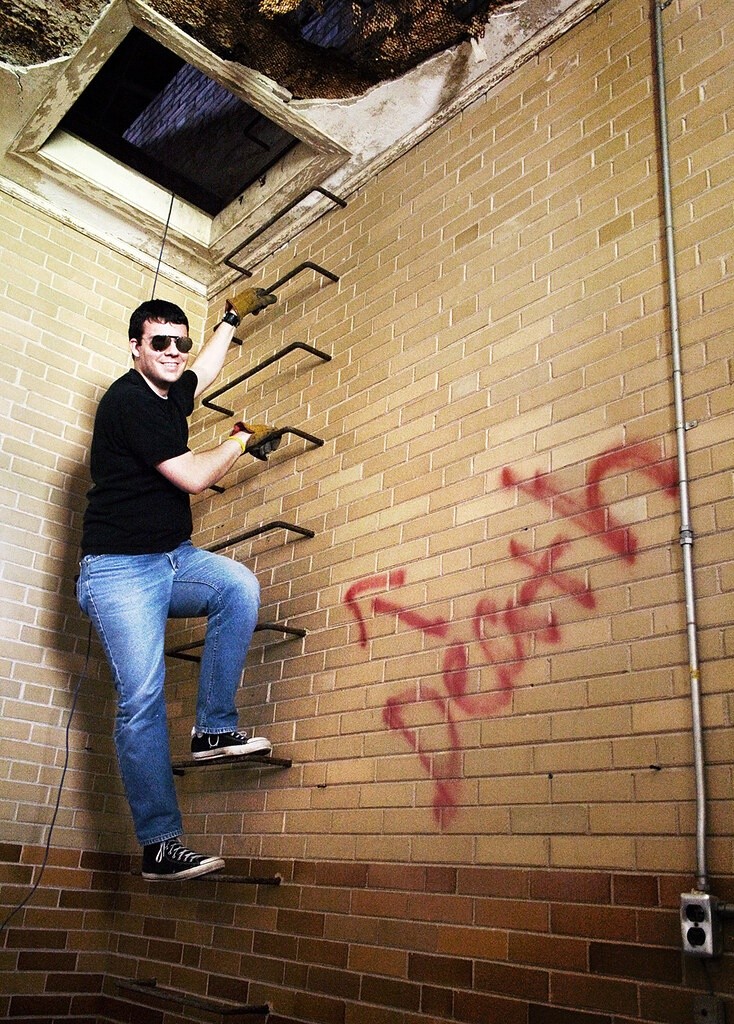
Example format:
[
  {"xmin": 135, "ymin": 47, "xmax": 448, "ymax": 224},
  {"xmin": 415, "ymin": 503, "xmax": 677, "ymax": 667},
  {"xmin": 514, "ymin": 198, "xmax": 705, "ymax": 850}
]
[
  {"xmin": 191, "ymin": 730, "xmax": 273, "ymax": 762},
  {"xmin": 142, "ymin": 834, "xmax": 225, "ymax": 883}
]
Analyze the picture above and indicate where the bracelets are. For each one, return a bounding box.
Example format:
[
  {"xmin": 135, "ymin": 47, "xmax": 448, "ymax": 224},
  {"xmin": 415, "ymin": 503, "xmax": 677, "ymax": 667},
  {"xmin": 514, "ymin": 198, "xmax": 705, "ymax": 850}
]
[
  {"xmin": 225, "ymin": 435, "xmax": 246, "ymax": 454},
  {"xmin": 222, "ymin": 310, "xmax": 240, "ymax": 328}
]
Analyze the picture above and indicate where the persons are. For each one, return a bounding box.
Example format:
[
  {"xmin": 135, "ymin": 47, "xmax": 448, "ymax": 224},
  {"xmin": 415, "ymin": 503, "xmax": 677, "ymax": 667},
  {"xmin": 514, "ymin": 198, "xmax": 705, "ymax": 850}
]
[{"xmin": 75, "ymin": 288, "xmax": 282, "ymax": 882}]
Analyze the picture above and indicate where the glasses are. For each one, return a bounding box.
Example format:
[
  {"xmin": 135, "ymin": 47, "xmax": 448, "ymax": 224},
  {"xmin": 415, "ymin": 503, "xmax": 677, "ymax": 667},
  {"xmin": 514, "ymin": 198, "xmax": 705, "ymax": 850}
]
[{"xmin": 135, "ymin": 335, "xmax": 192, "ymax": 353}]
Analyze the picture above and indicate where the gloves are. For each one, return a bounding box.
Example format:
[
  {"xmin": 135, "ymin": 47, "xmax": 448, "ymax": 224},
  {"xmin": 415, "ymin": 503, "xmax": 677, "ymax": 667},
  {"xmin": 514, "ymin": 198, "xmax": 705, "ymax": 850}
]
[
  {"xmin": 228, "ymin": 418, "xmax": 281, "ymax": 461},
  {"xmin": 225, "ymin": 287, "xmax": 278, "ymax": 325}
]
